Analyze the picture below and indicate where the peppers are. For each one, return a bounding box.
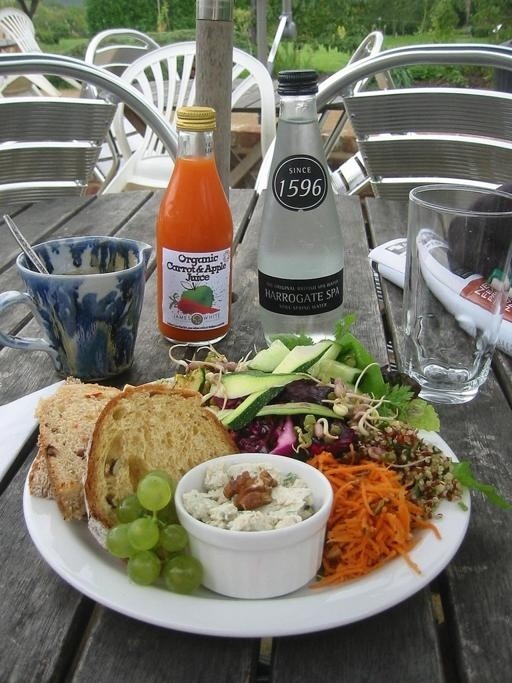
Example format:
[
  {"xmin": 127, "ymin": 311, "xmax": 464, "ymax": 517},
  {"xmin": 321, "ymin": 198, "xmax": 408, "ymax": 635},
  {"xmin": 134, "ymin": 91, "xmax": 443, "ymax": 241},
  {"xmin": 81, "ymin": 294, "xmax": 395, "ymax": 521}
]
[{"xmin": 170, "ymin": 293, "xmax": 219, "ymax": 314}]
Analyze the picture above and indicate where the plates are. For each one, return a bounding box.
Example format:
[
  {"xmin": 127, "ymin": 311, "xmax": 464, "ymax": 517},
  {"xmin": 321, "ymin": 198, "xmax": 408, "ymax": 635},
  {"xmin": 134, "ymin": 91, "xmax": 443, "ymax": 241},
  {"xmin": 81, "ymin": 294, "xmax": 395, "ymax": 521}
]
[{"xmin": 22, "ymin": 373, "xmax": 473, "ymax": 639}]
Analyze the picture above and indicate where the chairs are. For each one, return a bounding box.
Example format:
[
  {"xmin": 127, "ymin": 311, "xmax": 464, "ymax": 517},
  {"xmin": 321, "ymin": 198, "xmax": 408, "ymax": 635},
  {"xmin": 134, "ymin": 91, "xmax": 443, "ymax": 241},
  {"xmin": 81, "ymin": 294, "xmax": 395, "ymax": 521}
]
[
  {"xmin": 264, "ymin": 16, "xmax": 287, "ymax": 75},
  {"xmin": 77, "ymin": 29, "xmax": 181, "ymax": 194},
  {"xmin": 311, "ymin": 31, "xmax": 383, "ymax": 162},
  {"xmin": 254, "ymin": 43, "xmax": 511, "ymax": 199},
  {"xmin": 0, "ymin": 53, "xmax": 178, "ymax": 204},
  {"xmin": 0, "ymin": 6, "xmax": 82, "ymax": 97},
  {"xmin": 97, "ymin": 42, "xmax": 275, "ymax": 194}
]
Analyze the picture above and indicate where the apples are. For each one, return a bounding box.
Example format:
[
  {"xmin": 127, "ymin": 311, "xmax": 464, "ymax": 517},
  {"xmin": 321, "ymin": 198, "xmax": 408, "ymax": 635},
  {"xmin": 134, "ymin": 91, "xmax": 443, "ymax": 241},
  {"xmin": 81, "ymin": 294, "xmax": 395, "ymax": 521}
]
[{"xmin": 181, "ymin": 281, "xmax": 213, "ymax": 308}]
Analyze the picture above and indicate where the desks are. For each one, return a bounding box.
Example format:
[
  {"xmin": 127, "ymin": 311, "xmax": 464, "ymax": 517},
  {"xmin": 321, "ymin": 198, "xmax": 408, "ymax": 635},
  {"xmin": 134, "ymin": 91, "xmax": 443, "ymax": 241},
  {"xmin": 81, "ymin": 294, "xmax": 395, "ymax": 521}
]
[{"xmin": 98, "ymin": 79, "xmax": 344, "ymax": 189}]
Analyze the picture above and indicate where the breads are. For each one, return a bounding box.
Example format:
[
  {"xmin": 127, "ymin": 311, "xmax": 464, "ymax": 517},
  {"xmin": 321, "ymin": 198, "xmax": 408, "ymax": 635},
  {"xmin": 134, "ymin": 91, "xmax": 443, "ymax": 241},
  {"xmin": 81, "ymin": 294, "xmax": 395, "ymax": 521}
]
[{"xmin": 27, "ymin": 380, "xmax": 241, "ymax": 525}]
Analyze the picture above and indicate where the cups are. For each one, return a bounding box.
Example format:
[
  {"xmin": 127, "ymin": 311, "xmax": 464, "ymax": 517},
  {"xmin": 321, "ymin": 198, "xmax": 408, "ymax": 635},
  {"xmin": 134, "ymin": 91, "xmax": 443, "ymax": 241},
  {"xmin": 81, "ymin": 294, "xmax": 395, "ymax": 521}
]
[
  {"xmin": 169, "ymin": 452, "xmax": 336, "ymax": 598},
  {"xmin": 0, "ymin": 236, "xmax": 154, "ymax": 388},
  {"xmin": 395, "ymin": 183, "xmax": 511, "ymax": 404}
]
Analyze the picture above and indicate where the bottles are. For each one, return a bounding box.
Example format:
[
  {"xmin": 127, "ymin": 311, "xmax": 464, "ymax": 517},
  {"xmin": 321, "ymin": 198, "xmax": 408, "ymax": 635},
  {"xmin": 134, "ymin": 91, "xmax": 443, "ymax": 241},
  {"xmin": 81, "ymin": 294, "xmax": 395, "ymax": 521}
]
[
  {"xmin": 253, "ymin": 68, "xmax": 347, "ymax": 346},
  {"xmin": 154, "ymin": 106, "xmax": 236, "ymax": 344}
]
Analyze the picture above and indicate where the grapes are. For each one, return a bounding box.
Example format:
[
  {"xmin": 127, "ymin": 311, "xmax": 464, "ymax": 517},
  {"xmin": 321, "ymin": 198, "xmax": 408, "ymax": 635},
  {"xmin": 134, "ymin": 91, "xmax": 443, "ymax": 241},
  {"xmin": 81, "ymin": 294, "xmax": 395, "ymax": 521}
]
[{"xmin": 108, "ymin": 471, "xmax": 201, "ymax": 596}]
